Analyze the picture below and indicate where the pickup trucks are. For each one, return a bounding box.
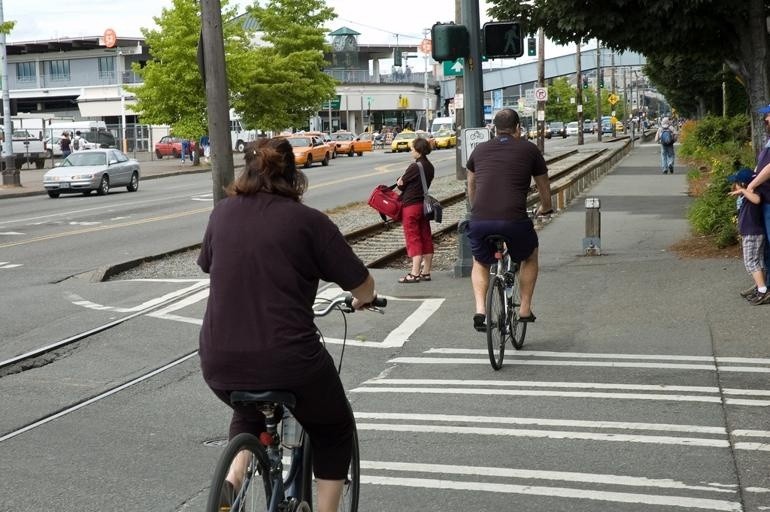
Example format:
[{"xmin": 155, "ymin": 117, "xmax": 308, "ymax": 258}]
[{"xmin": 0, "ymin": 124, "xmax": 47, "ymax": 169}]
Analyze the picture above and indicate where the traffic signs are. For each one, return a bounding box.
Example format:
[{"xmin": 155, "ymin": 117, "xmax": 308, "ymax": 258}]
[{"xmin": 443, "ymin": 58, "xmax": 464, "ymax": 77}]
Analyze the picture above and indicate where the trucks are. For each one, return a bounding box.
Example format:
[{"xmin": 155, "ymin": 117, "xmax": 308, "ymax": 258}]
[
  {"xmin": 430, "ymin": 117, "xmax": 456, "ymax": 136},
  {"xmin": 43, "ymin": 121, "xmax": 108, "ymax": 142},
  {"xmin": 229, "ymin": 108, "xmax": 272, "ymax": 153}
]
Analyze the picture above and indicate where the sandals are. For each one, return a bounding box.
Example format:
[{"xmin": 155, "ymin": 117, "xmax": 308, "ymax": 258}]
[{"xmin": 399, "ymin": 271, "xmax": 430, "ymax": 282}]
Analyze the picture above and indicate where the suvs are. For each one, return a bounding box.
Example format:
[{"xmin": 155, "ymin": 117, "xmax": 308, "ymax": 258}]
[{"xmin": 78, "ymin": 127, "xmax": 116, "ymax": 149}]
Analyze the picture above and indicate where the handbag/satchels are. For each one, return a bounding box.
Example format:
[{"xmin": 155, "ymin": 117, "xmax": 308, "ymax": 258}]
[
  {"xmin": 368, "ymin": 185, "xmax": 402, "ymax": 221},
  {"xmin": 423, "ymin": 195, "xmax": 442, "ymax": 222}
]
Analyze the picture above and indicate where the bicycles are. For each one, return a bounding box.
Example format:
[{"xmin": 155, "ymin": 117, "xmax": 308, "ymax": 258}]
[
  {"xmin": 205, "ymin": 296, "xmax": 388, "ymax": 512},
  {"xmin": 474, "ymin": 209, "xmax": 555, "ymax": 370}
]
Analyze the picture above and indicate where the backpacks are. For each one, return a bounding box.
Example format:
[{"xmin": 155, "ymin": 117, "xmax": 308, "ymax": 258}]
[{"xmin": 660, "ymin": 127, "xmax": 674, "ymax": 145}]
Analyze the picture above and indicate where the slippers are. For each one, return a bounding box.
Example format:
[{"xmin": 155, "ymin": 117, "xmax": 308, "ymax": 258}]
[
  {"xmin": 520, "ymin": 310, "xmax": 535, "ymax": 321},
  {"xmin": 474, "ymin": 314, "xmax": 485, "ymax": 328}
]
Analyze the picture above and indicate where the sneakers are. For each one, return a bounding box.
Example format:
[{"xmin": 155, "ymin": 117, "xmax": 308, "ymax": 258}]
[{"xmin": 741, "ymin": 284, "xmax": 770, "ymax": 305}]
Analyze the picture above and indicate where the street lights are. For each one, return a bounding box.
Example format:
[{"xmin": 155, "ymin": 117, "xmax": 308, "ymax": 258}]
[
  {"xmin": 583, "ymin": 77, "xmax": 588, "ymax": 89},
  {"xmin": 600, "ymin": 74, "xmax": 604, "ymax": 88},
  {"xmin": 527, "ymin": 38, "xmax": 536, "ymax": 57}
]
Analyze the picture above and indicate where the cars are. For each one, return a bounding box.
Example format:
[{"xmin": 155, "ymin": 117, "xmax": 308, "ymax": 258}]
[
  {"xmin": 275, "ymin": 129, "xmax": 373, "ymax": 168},
  {"xmin": 44, "ymin": 149, "xmax": 141, "ymax": 198},
  {"xmin": 44, "ymin": 136, "xmax": 102, "ymax": 158},
  {"xmin": 391, "ymin": 128, "xmax": 417, "ymax": 152},
  {"xmin": 529, "ymin": 116, "xmax": 654, "ymax": 139},
  {"xmin": 521, "ymin": 127, "xmax": 528, "ymax": 142},
  {"xmin": 433, "ymin": 127, "xmax": 457, "ymax": 150},
  {"xmin": 486, "ymin": 124, "xmax": 495, "ymax": 139},
  {"xmin": 415, "ymin": 130, "xmax": 437, "ymax": 152},
  {"xmin": 156, "ymin": 137, "xmax": 204, "ymax": 159}
]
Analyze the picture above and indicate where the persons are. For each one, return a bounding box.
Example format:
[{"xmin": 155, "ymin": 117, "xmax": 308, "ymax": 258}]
[
  {"xmin": 747, "ymin": 104, "xmax": 770, "ymax": 290},
  {"xmin": 72, "ymin": 131, "xmax": 84, "ymax": 153},
  {"xmin": 398, "ymin": 138, "xmax": 435, "ymax": 280},
  {"xmin": 182, "ymin": 121, "xmax": 414, "ymax": 163},
  {"xmin": 60, "ymin": 131, "xmax": 70, "ymax": 159},
  {"xmin": 656, "ymin": 117, "xmax": 677, "ymax": 174},
  {"xmin": 728, "ymin": 168, "xmax": 769, "ymax": 305},
  {"xmin": 198, "ymin": 137, "xmax": 374, "ymax": 511},
  {"xmin": 466, "ymin": 109, "xmax": 552, "ymax": 330}
]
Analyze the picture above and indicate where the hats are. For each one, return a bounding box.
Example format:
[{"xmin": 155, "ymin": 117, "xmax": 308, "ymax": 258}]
[{"xmin": 727, "ymin": 168, "xmax": 754, "ymax": 183}]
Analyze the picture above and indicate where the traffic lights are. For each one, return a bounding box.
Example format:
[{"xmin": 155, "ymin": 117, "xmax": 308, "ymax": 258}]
[{"xmin": 484, "ymin": 21, "xmax": 522, "ymax": 58}]
